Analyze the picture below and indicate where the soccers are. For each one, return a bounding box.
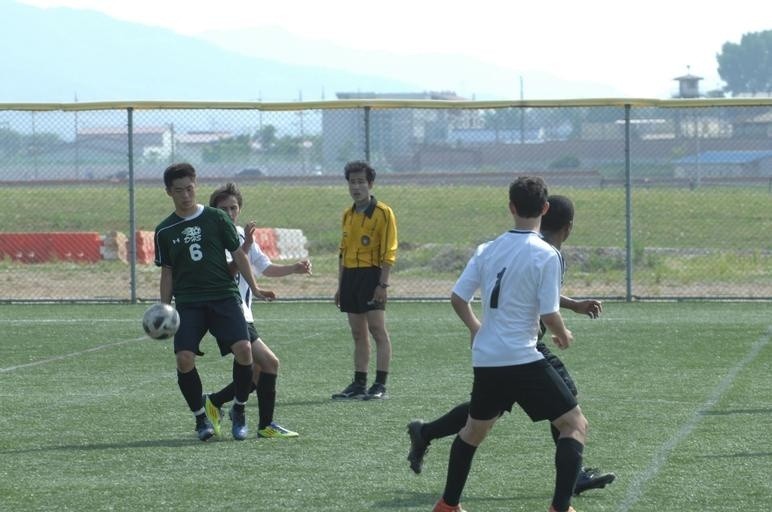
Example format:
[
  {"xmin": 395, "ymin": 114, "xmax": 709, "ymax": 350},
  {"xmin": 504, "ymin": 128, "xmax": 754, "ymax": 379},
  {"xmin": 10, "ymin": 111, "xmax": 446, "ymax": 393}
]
[{"xmin": 143, "ymin": 304, "xmax": 181, "ymax": 339}]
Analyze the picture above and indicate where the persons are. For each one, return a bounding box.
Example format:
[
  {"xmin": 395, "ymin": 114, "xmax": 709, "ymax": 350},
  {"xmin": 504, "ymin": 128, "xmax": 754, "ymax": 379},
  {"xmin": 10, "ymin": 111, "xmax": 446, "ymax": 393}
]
[
  {"xmin": 406, "ymin": 195, "xmax": 616, "ymax": 494},
  {"xmin": 432, "ymin": 173, "xmax": 590, "ymax": 512},
  {"xmin": 331, "ymin": 158, "xmax": 398, "ymax": 401},
  {"xmin": 202, "ymin": 179, "xmax": 313, "ymax": 438},
  {"xmin": 154, "ymin": 161, "xmax": 278, "ymax": 440}
]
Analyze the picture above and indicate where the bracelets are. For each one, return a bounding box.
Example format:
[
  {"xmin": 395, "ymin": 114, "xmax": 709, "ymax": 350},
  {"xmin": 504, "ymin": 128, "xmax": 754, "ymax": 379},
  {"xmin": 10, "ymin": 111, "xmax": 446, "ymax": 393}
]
[{"xmin": 378, "ymin": 280, "xmax": 389, "ymax": 289}]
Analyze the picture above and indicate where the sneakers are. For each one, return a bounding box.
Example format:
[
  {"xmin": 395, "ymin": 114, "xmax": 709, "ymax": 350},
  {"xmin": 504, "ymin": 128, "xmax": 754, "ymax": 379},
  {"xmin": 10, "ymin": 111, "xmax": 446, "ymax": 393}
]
[
  {"xmin": 575, "ymin": 466, "xmax": 615, "ymax": 495},
  {"xmin": 332, "ymin": 379, "xmax": 386, "ymax": 400},
  {"xmin": 257, "ymin": 421, "xmax": 299, "ymax": 438},
  {"xmin": 407, "ymin": 422, "xmax": 430, "ymax": 474},
  {"xmin": 195, "ymin": 394, "xmax": 248, "ymax": 440}
]
